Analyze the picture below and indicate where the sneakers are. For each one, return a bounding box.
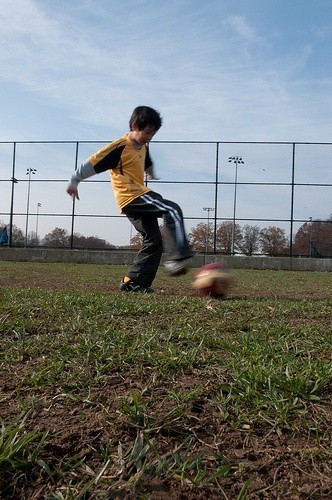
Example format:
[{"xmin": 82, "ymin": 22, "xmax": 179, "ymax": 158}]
[{"xmin": 119, "ymin": 278, "xmax": 154, "ymax": 295}]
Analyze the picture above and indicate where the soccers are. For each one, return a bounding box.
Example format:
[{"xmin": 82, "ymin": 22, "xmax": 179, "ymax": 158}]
[{"xmin": 193, "ymin": 262, "xmax": 232, "ymax": 298}]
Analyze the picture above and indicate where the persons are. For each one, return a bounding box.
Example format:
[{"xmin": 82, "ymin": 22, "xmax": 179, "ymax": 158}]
[{"xmin": 65, "ymin": 104, "xmax": 197, "ymax": 293}]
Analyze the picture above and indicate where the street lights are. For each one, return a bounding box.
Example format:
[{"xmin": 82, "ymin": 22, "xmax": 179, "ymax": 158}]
[
  {"xmin": 203, "ymin": 207, "xmax": 214, "ymax": 254},
  {"xmin": 24, "ymin": 168, "xmax": 38, "ymax": 246},
  {"xmin": 35, "ymin": 202, "xmax": 41, "ymax": 247},
  {"xmin": 228, "ymin": 155, "xmax": 244, "ymax": 256}
]
